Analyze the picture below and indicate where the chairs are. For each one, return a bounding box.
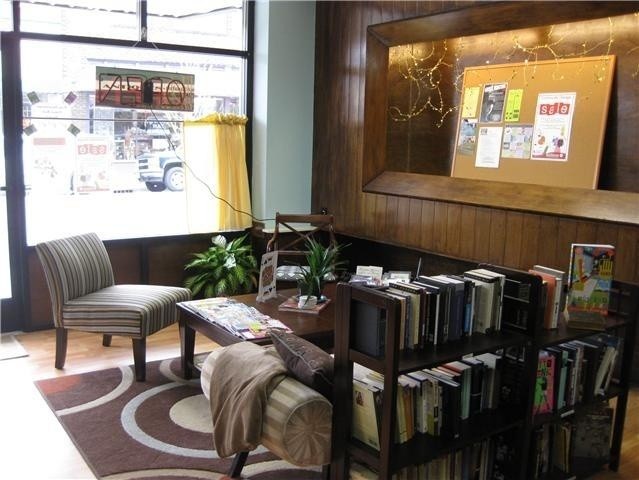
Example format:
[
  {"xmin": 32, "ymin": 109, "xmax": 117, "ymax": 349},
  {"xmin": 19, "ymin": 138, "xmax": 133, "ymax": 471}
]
[
  {"xmin": 36, "ymin": 233, "xmax": 193, "ymax": 382},
  {"xmin": 267, "ymin": 211, "xmax": 339, "ymax": 289}
]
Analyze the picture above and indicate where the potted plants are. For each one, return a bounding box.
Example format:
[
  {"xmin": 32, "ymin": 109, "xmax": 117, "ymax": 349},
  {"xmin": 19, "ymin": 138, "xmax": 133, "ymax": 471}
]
[{"xmin": 293, "ymin": 237, "xmax": 352, "ymax": 305}]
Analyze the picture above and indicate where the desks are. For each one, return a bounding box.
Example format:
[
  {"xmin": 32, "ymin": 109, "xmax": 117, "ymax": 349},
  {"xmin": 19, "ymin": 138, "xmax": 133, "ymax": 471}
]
[{"xmin": 176, "ymin": 283, "xmax": 336, "ymax": 380}]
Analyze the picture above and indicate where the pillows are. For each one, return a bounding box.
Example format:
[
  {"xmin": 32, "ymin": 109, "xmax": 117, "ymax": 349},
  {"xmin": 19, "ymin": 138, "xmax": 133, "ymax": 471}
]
[{"xmin": 268, "ymin": 327, "xmax": 334, "ymax": 405}]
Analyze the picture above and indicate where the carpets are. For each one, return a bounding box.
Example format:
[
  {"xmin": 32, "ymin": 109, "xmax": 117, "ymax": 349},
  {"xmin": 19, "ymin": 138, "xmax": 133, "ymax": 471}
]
[
  {"xmin": 0, "ymin": 333, "xmax": 29, "ymax": 361},
  {"xmin": 34, "ymin": 351, "xmax": 328, "ymax": 480}
]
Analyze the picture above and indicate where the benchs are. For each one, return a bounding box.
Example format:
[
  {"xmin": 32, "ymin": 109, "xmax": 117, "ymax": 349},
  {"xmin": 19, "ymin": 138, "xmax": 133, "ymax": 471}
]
[{"xmin": 200, "ymin": 341, "xmax": 334, "ymax": 480}]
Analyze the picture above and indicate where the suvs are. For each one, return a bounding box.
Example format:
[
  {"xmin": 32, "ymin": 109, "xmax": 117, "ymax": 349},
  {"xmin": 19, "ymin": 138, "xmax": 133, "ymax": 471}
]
[{"xmin": 137, "ymin": 142, "xmax": 185, "ymax": 193}]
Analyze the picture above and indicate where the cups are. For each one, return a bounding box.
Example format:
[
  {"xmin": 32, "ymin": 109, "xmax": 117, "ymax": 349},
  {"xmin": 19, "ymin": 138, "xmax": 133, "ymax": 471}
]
[{"xmin": 298, "ymin": 295, "xmax": 317, "ymax": 311}]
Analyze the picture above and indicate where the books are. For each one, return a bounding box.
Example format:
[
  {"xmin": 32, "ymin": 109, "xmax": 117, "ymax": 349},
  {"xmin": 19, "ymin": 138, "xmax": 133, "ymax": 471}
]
[
  {"xmin": 330, "ymin": 333, "xmax": 623, "ymax": 452},
  {"xmin": 348, "ymin": 244, "xmax": 615, "ymax": 357},
  {"xmin": 349, "ymin": 400, "xmax": 614, "ymax": 479},
  {"xmin": 175, "ymin": 297, "xmax": 293, "ymax": 340}
]
